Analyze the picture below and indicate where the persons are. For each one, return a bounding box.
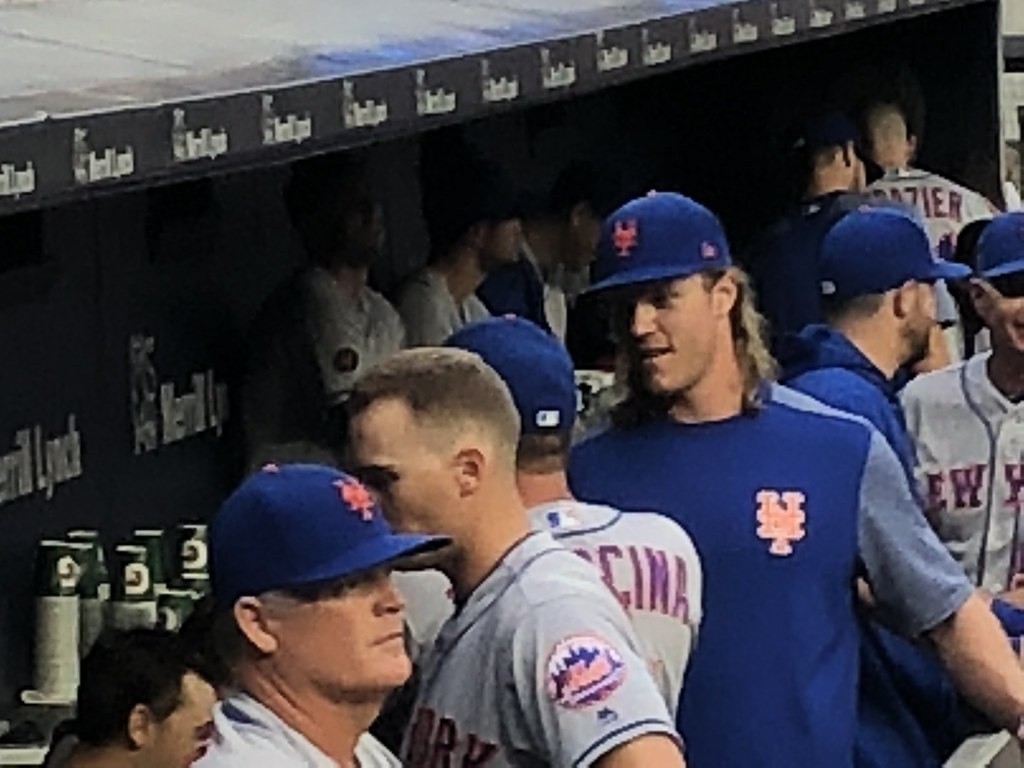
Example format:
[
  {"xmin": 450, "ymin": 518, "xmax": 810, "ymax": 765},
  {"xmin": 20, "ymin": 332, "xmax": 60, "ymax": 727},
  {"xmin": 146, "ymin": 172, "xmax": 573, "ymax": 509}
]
[{"xmin": 42, "ymin": 92, "xmax": 1024, "ymax": 768}]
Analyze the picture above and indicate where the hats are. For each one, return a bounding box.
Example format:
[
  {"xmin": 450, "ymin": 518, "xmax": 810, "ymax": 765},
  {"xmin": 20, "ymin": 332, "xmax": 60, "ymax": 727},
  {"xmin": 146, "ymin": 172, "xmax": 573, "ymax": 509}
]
[
  {"xmin": 583, "ymin": 192, "xmax": 730, "ymax": 297},
  {"xmin": 976, "ymin": 212, "xmax": 1024, "ymax": 278},
  {"xmin": 208, "ymin": 461, "xmax": 453, "ymax": 597},
  {"xmin": 822, "ymin": 203, "xmax": 973, "ymax": 297},
  {"xmin": 441, "ymin": 315, "xmax": 579, "ymax": 440},
  {"xmin": 782, "ymin": 114, "xmax": 886, "ymax": 188}
]
